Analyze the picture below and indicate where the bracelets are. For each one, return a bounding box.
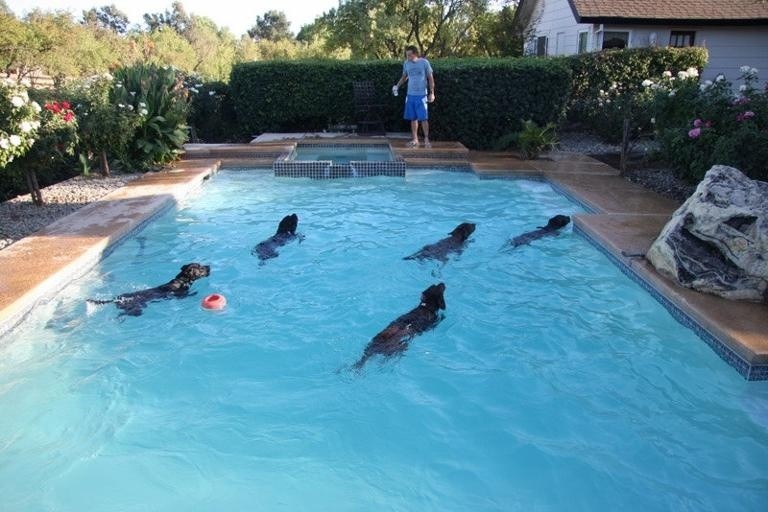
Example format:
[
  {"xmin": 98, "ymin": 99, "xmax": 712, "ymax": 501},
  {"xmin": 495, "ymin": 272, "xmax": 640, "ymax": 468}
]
[{"xmin": 430, "ymin": 90, "xmax": 435, "ymax": 94}]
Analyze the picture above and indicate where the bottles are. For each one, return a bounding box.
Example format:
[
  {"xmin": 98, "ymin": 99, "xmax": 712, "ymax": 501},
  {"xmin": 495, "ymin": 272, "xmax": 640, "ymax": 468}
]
[{"xmin": 393, "ymin": 85, "xmax": 398, "ymax": 97}]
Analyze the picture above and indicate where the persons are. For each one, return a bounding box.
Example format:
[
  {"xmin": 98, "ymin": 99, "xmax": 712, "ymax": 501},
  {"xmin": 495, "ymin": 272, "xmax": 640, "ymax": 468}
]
[{"xmin": 392, "ymin": 45, "xmax": 435, "ymax": 145}]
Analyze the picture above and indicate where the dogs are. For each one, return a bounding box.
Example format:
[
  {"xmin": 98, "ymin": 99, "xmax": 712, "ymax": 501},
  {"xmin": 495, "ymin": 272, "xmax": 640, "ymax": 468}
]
[
  {"xmin": 248, "ymin": 212, "xmax": 307, "ymax": 267},
  {"xmin": 354, "ymin": 282, "xmax": 447, "ymax": 371},
  {"xmin": 507, "ymin": 213, "xmax": 571, "ymax": 249},
  {"xmin": 401, "ymin": 222, "xmax": 478, "ymax": 272},
  {"xmin": 85, "ymin": 261, "xmax": 214, "ymax": 312}
]
